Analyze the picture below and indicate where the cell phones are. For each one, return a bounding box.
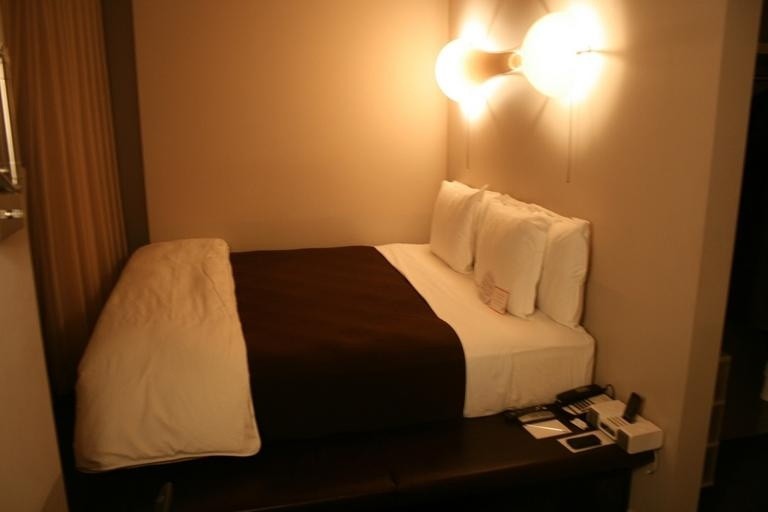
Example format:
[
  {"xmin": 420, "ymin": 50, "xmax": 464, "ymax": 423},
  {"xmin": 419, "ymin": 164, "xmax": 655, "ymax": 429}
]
[
  {"xmin": 558, "ymin": 383, "xmax": 606, "ymax": 402},
  {"xmin": 566, "ymin": 435, "xmax": 600, "ymax": 449},
  {"xmin": 621, "ymin": 392, "xmax": 639, "ymax": 423}
]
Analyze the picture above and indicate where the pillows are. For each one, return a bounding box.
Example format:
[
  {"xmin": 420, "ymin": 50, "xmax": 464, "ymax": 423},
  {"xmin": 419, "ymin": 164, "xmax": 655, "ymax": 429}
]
[{"xmin": 431, "ymin": 180, "xmax": 591, "ymax": 328}]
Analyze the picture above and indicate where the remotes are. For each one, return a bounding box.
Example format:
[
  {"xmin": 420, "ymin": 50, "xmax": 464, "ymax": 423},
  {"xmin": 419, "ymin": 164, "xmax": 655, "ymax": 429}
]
[
  {"xmin": 517, "ymin": 411, "xmax": 555, "ymax": 423},
  {"xmin": 503, "ymin": 405, "xmax": 549, "ymax": 420}
]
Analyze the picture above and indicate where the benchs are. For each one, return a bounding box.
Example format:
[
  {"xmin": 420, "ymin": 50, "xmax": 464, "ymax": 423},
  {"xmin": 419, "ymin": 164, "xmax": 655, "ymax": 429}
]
[{"xmin": 212, "ymin": 407, "xmax": 652, "ymax": 512}]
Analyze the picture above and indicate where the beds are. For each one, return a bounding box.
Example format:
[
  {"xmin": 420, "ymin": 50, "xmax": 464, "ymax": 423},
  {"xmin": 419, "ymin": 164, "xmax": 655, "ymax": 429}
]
[{"xmin": 74, "ymin": 238, "xmax": 599, "ymax": 472}]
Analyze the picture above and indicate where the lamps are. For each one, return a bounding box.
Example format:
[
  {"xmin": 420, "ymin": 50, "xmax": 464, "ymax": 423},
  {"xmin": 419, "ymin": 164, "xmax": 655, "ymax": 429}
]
[{"xmin": 434, "ymin": 8, "xmax": 609, "ymax": 112}]
